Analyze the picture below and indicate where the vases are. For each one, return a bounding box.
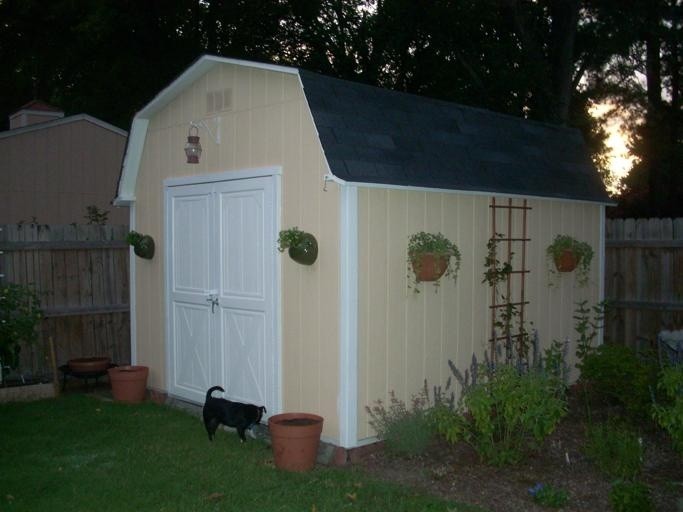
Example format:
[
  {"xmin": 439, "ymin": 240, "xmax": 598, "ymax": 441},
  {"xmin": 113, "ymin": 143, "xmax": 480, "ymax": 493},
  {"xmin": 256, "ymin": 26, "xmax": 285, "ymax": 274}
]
[
  {"xmin": 67, "ymin": 356, "xmax": 111, "ymax": 374},
  {"xmin": 108, "ymin": 364, "xmax": 150, "ymax": 407}
]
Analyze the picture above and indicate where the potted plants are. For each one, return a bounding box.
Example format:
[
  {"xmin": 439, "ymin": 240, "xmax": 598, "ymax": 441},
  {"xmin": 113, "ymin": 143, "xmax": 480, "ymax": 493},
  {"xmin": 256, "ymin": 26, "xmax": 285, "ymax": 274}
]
[
  {"xmin": 543, "ymin": 231, "xmax": 594, "ymax": 293},
  {"xmin": 274, "ymin": 226, "xmax": 318, "ymax": 266},
  {"xmin": 0, "ymin": 274, "xmax": 60, "ymax": 405},
  {"xmin": 404, "ymin": 231, "xmax": 461, "ymax": 298},
  {"xmin": 124, "ymin": 226, "xmax": 154, "ymax": 262}
]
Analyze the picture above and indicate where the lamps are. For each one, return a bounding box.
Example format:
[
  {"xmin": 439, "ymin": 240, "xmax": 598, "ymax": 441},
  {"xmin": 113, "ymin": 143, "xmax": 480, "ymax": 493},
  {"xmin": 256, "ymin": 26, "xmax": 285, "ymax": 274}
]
[{"xmin": 182, "ymin": 123, "xmax": 202, "ymax": 165}]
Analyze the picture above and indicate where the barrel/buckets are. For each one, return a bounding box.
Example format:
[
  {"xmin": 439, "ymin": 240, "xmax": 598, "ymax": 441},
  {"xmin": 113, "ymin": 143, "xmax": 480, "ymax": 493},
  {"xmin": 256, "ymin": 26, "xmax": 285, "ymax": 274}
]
[{"xmin": 106, "ymin": 365, "xmax": 150, "ymax": 402}]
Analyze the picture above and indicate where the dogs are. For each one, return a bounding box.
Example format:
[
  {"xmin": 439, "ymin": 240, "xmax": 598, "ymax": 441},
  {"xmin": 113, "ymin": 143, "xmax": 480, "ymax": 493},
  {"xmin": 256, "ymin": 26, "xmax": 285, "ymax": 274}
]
[{"xmin": 202, "ymin": 385, "xmax": 267, "ymax": 443}]
